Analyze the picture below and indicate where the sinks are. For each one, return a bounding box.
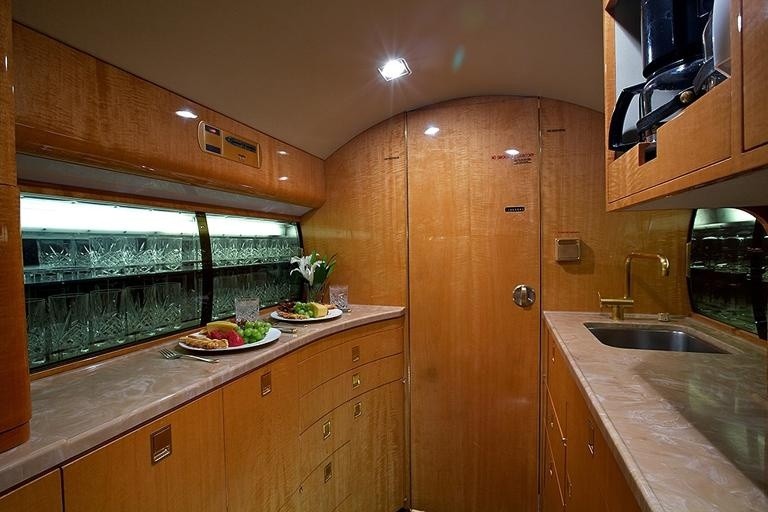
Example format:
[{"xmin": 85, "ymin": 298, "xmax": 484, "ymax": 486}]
[{"xmin": 585, "ymin": 325, "xmax": 733, "ymax": 355}]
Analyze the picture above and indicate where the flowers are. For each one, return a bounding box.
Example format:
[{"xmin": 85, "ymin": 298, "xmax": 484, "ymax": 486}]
[{"xmin": 290, "ymin": 249, "xmax": 338, "ymax": 294}]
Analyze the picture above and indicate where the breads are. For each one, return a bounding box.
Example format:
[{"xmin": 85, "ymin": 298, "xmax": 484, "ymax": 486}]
[
  {"xmin": 179, "ymin": 334, "xmax": 228, "ymax": 349},
  {"xmin": 279, "ymin": 312, "xmax": 307, "ymax": 320}
]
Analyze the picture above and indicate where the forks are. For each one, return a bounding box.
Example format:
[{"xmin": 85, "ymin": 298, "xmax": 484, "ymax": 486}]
[{"xmin": 159, "ymin": 348, "xmax": 219, "ymax": 364}]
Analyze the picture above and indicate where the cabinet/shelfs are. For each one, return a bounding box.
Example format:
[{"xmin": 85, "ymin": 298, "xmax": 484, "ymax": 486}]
[
  {"xmin": 298, "ymin": 315, "xmax": 411, "ymax": 512},
  {"xmin": 0, "ymin": 467, "xmax": 62, "ymax": 511},
  {"xmin": 566, "ymin": 356, "xmax": 640, "ymax": 512},
  {"xmin": 541, "ymin": 331, "xmax": 566, "ymax": 512},
  {"xmin": 729, "ymin": 0, "xmax": 768, "ymax": 175},
  {"xmin": 222, "ymin": 350, "xmax": 298, "ymax": 512},
  {"xmin": 62, "ymin": 388, "xmax": 226, "ymax": 511}
]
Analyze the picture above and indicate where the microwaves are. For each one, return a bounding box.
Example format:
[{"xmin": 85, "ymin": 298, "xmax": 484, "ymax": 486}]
[{"xmin": 684, "ymin": 204, "xmax": 768, "ymax": 340}]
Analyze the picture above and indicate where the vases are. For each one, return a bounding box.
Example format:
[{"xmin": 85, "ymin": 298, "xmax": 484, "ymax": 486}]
[{"xmin": 307, "ymin": 286, "xmax": 325, "ymax": 304}]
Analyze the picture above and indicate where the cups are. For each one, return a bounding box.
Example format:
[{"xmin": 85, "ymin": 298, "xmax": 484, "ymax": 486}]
[
  {"xmin": 199, "ymin": 277, "xmax": 219, "ymax": 320},
  {"xmin": 166, "ymin": 288, "xmax": 186, "ymax": 323},
  {"xmin": 265, "ymin": 271, "xmax": 278, "ymax": 306},
  {"xmin": 218, "ymin": 275, "xmax": 238, "ymax": 316},
  {"xmin": 185, "ymin": 237, "xmax": 300, "ymax": 266},
  {"xmin": 92, "ymin": 236, "xmax": 184, "ymax": 272},
  {"xmin": 238, "ymin": 273, "xmax": 256, "ymax": 300},
  {"xmin": 277, "ymin": 270, "xmax": 290, "ymax": 304},
  {"xmin": 90, "ymin": 289, "xmax": 127, "ymax": 347},
  {"xmin": 152, "ymin": 282, "xmax": 182, "ymax": 332},
  {"xmin": 288, "ymin": 269, "xmax": 301, "ymax": 298},
  {"xmin": 61, "ymin": 240, "xmax": 76, "ymax": 266},
  {"xmin": 250, "ymin": 272, "xmax": 266, "ymax": 307},
  {"xmin": 124, "ymin": 285, "xmax": 157, "ymax": 337},
  {"xmin": 48, "ymin": 294, "xmax": 91, "ymax": 357},
  {"xmin": 24, "ymin": 298, "xmax": 47, "ymax": 364},
  {"xmin": 329, "ymin": 285, "xmax": 348, "ymax": 310},
  {"xmin": 235, "ymin": 297, "xmax": 259, "ymax": 324},
  {"xmin": 36, "ymin": 239, "xmax": 64, "ymax": 266},
  {"xmin": 23, "ymin": 266, "xmax": 96, "ymax": 283},
  {"xmin": 75, "ymin": 238, "xmax": 92, "ymax": 267},
  {"xmin": 185, "ymin": 289, "xmax": 197, "ymax": 321}
]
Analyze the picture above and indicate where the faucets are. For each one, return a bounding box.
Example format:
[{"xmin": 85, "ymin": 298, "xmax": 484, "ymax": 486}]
[{"xmin": 622, "ymin": 251, "xmax": 671, "ymax": 300}]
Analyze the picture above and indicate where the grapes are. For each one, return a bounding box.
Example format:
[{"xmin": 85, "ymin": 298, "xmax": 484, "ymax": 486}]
[
  {"xmin": 229, "ymin": 317, "xmax": 246, "ymax": 328},
  {"xmin": 293, "ymin": 301, "xmax": 313, "ymax": 317},
  {"xmin": 239, "ymin": 322, "xmax": 271, "ymax": 342},
  {"xmin": 279, "ymin": 301, "xmax": 294, "ymax": 312}
]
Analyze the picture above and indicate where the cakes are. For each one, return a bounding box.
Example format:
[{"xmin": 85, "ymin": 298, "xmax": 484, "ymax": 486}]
[
  {"xmin": 311, "ymin": 300, "xmax": 328, "ymax": 318},
  {"xmin": 207, "ymin": 321, "xmax": 238, "ymax": 334}
]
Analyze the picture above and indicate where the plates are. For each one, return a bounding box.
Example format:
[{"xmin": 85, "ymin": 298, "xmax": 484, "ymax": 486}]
[
  {"xmin": 178, "ymin": 328, "xmax": 281, "ymax": 351},
  {"xmin": 271, "ymin": 308, "xmax": 343, "ymax": 321}
]
